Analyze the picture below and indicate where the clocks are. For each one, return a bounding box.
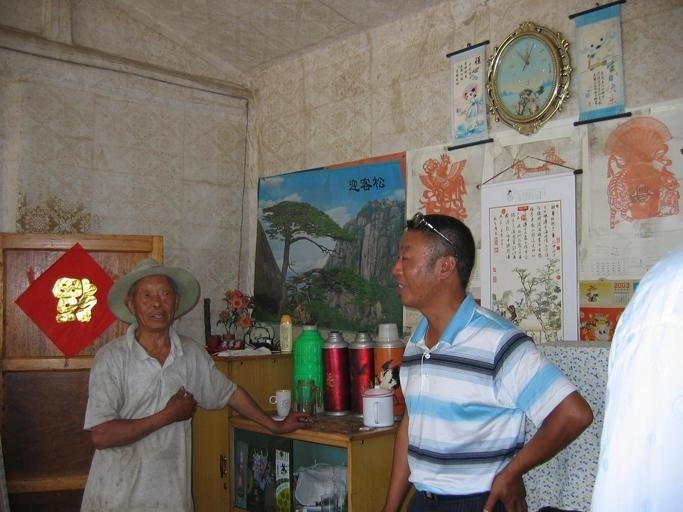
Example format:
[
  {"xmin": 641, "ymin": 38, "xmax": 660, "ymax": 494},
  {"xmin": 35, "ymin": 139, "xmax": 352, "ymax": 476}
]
[{"xmin": 486, "ymin": 21, "xmax": 574, "ymax": 135}]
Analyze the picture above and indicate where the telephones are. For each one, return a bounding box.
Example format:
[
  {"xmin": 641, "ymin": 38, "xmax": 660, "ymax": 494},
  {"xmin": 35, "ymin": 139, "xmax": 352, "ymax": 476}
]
[{"xmin": 206, "ymin": 334, "xmax": 245, "ymax": 353}]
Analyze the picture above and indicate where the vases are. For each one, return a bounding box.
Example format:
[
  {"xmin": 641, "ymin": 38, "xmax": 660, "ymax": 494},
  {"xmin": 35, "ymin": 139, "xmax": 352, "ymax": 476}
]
[{"xmin": 223, "ymin": 335, "xmax": 235, "ymax": 340}]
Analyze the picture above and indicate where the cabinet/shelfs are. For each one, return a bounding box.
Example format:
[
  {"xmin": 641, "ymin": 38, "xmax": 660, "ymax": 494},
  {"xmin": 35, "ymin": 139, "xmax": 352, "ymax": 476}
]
[
  {"xmin": 228, "ymin": 411, "xmax": 416, "ymax": 512},
  {"xmin": 192, "ymin": 346, "xmax": 293, "ymax": 512}
]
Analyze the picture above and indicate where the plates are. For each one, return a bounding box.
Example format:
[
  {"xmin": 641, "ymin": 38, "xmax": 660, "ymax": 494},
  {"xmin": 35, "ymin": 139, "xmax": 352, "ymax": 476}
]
[{"xmin": 247, "ymin": 321, "xmax": 274, "ymax": 346}]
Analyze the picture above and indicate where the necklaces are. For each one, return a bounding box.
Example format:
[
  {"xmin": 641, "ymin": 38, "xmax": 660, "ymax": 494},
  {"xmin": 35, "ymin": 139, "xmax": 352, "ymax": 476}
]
[{"xmin": 424, "ymin": 323, "xmax": 432, "ymax": 350}]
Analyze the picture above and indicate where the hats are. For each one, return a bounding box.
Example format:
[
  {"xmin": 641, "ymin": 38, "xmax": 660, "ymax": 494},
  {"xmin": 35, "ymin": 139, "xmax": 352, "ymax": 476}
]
[{"xmin": 108, "ymin": 258, "xmax": 201, "ymax": 324}]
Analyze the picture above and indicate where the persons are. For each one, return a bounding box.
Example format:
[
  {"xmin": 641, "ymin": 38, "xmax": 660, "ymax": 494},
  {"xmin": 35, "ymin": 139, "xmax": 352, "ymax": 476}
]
[
  {"xmin": 76, "ymin": 255, "xmax": 313, "ymax": 511},
  {"xmin": 592, "ymin": 247, "xmax": 683, "ymax": 512},
  {"xmin": 381, "ymin": 209, "xmax": 594, "ymax": 511}
]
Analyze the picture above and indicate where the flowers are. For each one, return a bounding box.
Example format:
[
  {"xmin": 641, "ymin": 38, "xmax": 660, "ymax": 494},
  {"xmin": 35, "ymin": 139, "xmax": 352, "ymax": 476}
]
[{"xmin": 217, "ymin": 290, "xmax": 255, "ymax": 335}]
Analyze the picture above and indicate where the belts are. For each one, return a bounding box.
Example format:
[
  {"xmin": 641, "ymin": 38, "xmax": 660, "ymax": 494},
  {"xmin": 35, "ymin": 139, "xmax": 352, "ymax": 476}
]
[{"xmin": 423, "ymin": 491, "xmax": 488, "ymax": 501}]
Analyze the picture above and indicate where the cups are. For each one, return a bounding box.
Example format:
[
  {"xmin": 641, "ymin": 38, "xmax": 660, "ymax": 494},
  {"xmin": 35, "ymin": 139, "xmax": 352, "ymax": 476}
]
[
  {"xmin": 361, "ymin": 390, "xmax": 395, "ymax": 428},
  {"xmin": 297, "ymin": 379, "xmax": 323, "ymax": 417},
  {"xmin": 321, "ymin": 494, "xmax": 337, "ymax": 511},
  {"xmin": 269, "ymin": 390, "xmax": 291, "ymax": 418}
]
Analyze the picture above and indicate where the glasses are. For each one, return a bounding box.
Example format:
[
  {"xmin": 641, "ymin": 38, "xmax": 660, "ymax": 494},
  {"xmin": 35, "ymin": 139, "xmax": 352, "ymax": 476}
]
[{"xmin": 411, "ymin": 212, "xmax": 458, "ymax": 257}]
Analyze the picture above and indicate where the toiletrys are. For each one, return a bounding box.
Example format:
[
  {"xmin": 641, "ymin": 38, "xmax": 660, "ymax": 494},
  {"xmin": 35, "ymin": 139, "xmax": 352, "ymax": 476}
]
[{"xmin": 279, "ymin": 314, "xmax": 293, "ymax": 353}]
